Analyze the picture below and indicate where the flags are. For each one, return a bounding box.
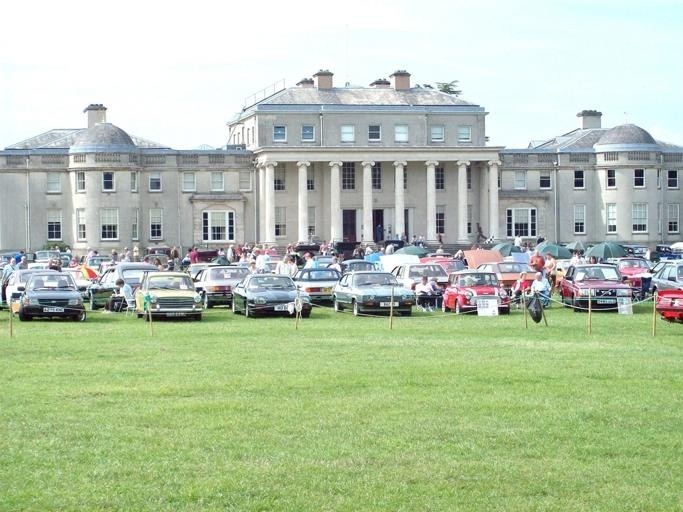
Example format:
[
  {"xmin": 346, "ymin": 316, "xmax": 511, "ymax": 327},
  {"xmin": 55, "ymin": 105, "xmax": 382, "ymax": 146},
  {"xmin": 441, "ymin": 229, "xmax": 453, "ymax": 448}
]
[{"xmin": 81, "ymin": 265, "xmax": 99, "ymax": 279}]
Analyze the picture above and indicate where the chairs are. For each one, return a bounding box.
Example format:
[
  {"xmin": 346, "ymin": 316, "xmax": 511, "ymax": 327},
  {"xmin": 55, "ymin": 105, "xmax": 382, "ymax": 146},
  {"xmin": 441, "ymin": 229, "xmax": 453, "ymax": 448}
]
[
  {"xmin": 633, "ymin": 277, "xmax": 652, "ymax": 302},
  {"xmin": 416, "ymin": 291, "xmax": 437, "ymax": 311},
  {"xmin": 509, "ymin": 287, "xmax": 527, "ymax": 309},
  {"xmin": 527, "ymin": 289, "xmax": 551, "ymax": 308},
  {"xmin": 115, "ymin": 284, "xmax": 140, "ymax": 312}
]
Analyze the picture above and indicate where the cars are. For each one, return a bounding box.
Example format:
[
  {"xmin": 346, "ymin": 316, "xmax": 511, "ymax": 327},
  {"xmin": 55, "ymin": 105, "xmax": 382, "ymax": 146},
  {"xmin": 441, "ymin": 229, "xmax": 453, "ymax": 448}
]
[
  {"xmin": 331, "ymin": 271, "xmax": 413, "ymax": 316},
  {"xmin": 17, "ymin": 273, "xmax": 86, "ymax": 322},
  {"xmin": 135, "ymin": 272, "xmax": 202, "ymax": 321},
  {"xmin": 647, "ymin": 259, "xmax": 683, "ymax": 292},
  {"xmin": 654, "ymin": 289, "xmax": 682, "ymax": 324},
  {"xmin": 0, "ymin": 241, "xmax": 595, "ymax": 301},
  {"xmin": 230, "ymin": 273, "xmax": 311, "ymax": 317},
  {"xmin": 606, "ymin": 257, "xmax": 653, "ymax": 295},
  {"xmin": 441, "ymin": 269, "xmax": 509, "ymax": 315},
  {"xmin": 558, "ymin": 264, "xmax": 631, "ymax": 311}
]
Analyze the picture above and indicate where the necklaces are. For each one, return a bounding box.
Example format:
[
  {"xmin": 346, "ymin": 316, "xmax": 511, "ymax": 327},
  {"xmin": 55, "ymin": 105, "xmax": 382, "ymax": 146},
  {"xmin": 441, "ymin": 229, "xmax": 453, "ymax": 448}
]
[{"xmin": 218, "ymin": 241, "xmax": 314, "ymax": 280}]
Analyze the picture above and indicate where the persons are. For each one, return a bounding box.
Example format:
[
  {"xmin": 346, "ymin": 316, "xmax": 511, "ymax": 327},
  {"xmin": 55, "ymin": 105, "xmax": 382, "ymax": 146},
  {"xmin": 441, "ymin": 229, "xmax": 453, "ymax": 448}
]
[
  {"xmin": 528, "ymin": 250, "xmax": 557, "ymax": 292},
  {"xmin": 400, "ymin": 233, "xmax": 464, "ymax": 261},
  {"xmin": 514, "ymin": 234, "xmax": 545, "ymax": 252},
  {"xmin": 415, "ymin": 276, "xmax": 444, "ymax": 312},
  {"xmin": 0, "ymin": 250, "xmax": 28, "ymax": 306},
  {"xmin": 48, "ymin": 246, "xmax": 99, "ymax": 274},
  {"xmin": 319, "ymin": 225, "xmax": 396, "ymax": 279},
  {"xmin": 509, "ymin": 271, "xmax": 551, "ymax": 310},
  {"xmin": 570, "ymin": 251, "xmax": 595, "ymax": 265},
  {"xmin": 470, "ymin": 222, "xmax": 488, "ymax": 250},
  {"xmin": 643, "ymin": 248, "xmax": 653, "ymax": 261},
  {"xmin": 105, "ymin": 278, "xmax": 134, "ymax": 312},
  {"xmin": 111, "ymin": 245, "xmax": 199, "ymax": 273}
]
